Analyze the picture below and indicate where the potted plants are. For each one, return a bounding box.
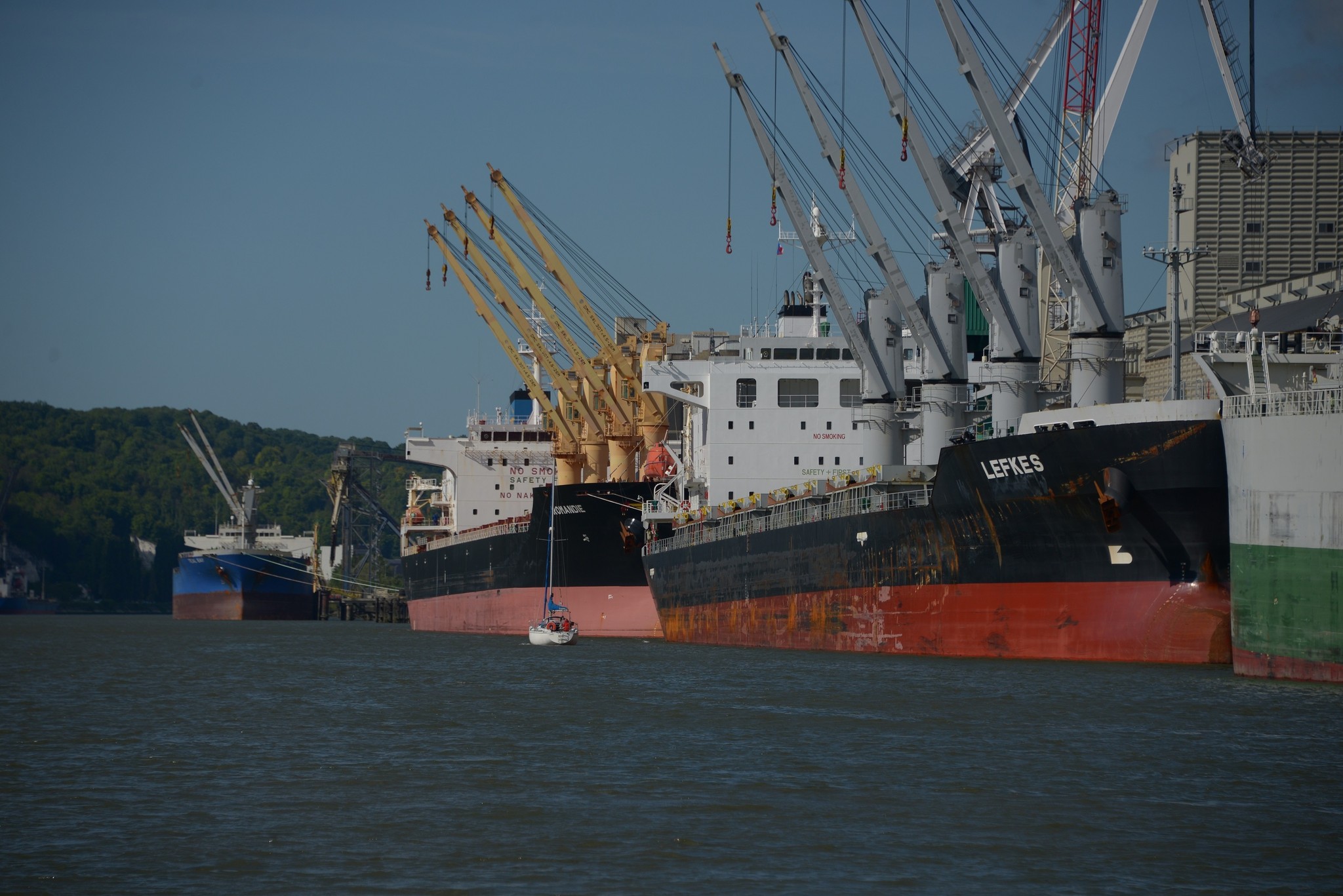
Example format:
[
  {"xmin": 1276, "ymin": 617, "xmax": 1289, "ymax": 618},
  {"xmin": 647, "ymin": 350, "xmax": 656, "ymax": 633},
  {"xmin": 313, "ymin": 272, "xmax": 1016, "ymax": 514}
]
[
  {"xmin": 797, "ymin": 510, "xmax": 802, "ymax": 525},
  {"xmin": 742, "ymin": 524, "xmax": 747, "ymax": 536},
  {"xmin": 862, "ymin": 498, "xmax": 871, "ymax": 513}
]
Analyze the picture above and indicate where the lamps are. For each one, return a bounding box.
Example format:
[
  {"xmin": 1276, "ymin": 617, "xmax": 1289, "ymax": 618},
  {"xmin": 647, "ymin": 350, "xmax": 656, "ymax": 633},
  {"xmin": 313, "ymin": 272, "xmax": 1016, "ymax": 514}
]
[
  {"xmin": 986, "ymin": 307, "xmax": 990, "ymax": 311},
  {"xmin": 948, "ymin": 434, "xmax": 964, "ymax": 445},
  {"xmin": 657, "ymin": 360, "xmax": 662, "ymax": 365},
  {"xmin": 668, "ymin": 361, "xmax": 673, "ymax": 366},
  {"xmin": 984, "ymin": 364, "xmax": 989, "ymax": 368},
  {"xmin": 637, "ymin": 495, "xmax": 644, "ymax": 503},
  {"xmin": 964, "ymin": 431, "xmax": 975, "ymax": 442}
]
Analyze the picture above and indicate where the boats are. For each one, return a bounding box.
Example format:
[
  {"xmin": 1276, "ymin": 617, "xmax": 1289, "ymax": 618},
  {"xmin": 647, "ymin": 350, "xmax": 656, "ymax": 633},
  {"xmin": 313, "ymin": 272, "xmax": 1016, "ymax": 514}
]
[
  {"xmin": 318, "ymin": 441, "xmax": 411, "ymax": 622},
  {"xmin": 528, "ymin": 455, "xmax": 578, "ymax": 645},
  {"xmin": 640, "ymin": 0, "xmax": 1235, "ymax": 666},
  {"xmin": 172, "ymin": 408, "xmax": 325, "ymax": 620},
  {"xmin": 400, "ymin": 158, "xmax": 704, "ymax": 639}
]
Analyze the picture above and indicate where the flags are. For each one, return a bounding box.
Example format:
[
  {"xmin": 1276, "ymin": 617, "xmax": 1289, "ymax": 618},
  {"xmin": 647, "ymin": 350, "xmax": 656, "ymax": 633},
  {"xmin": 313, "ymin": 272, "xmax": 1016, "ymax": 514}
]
[{"xmin": 777, "ymin": 243, "xmax": 783, "ymax": 254}]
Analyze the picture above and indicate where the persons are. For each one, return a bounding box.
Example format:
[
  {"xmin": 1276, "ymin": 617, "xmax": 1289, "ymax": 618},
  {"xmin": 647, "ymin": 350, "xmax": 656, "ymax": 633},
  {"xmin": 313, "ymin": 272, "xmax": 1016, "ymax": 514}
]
[
  {"xmin": 563, "ymin": 619, "xmax": 569, "ymax": 631},
  {"xmin": 432, "ymin": 512, "xmax": 440, "ymax": 525}
]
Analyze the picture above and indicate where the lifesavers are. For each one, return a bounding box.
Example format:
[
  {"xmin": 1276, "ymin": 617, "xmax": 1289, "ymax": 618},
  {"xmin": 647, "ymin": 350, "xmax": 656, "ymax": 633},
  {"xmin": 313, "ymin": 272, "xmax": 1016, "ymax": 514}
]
[
  {"xmin": 680, "ymin": 500, "xmax": 690, "ymax": 510},
  {"xmin": 546, "ymin": 622, "xmax": 556, "ymax": 630}
]
